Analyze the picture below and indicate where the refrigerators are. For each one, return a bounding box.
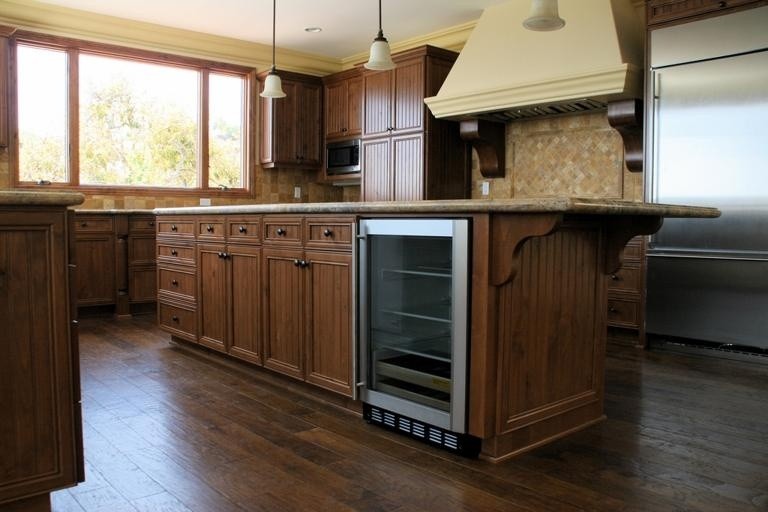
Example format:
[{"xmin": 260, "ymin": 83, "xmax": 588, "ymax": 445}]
[{"xmin": 646, "ymin": 6, "xmax": 768, "ymax": 365}]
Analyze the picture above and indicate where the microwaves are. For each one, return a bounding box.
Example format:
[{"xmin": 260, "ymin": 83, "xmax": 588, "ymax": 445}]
[{"xmin": 323, "ymin": 138, "xmax": 360, "ymax": 174}]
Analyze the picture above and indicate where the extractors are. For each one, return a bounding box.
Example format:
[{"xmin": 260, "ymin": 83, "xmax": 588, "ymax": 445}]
[{"xmin": 424, "ymin": 0, "xmax": 646, "ymax": 121}]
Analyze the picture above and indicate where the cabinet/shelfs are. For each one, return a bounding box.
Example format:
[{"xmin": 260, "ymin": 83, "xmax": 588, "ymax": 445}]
[
  {"xmin": 156, "ymin": 212, "xmax": 662, "ymax": 465},
  {"xmin": 72, "ymin": 214, "xmax": 154, "ymax": 315},
  {"xmin": 318, "ymin": 58, "xmax": 365, "ymax": 182},
  {"xmin": 607, "ymin": 234, "xmax": 651, "ymax": 349},
  {"xmin": 260, "ymin": 82, "xmax": 322, "ymax": 168},
  {"xmin": 360, "ymin": 60, "xmax": 469, "ymax": 199},
  {"xmin": 0, "ymin": 206, "xmax": 85, "ymax": 512}
]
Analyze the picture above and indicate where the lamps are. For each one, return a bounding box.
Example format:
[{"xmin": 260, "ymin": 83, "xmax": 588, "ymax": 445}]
[
  {"xmin": 522, "ymin": 1, "xmax": 565, "ymax": 30},
  {"xmin": 364, "ymin": 0, "xmax": 397, "ymax": 70},
  {"xmin": 261, "ymin": 1, "xmax": 287, "ymax": 99}
]
